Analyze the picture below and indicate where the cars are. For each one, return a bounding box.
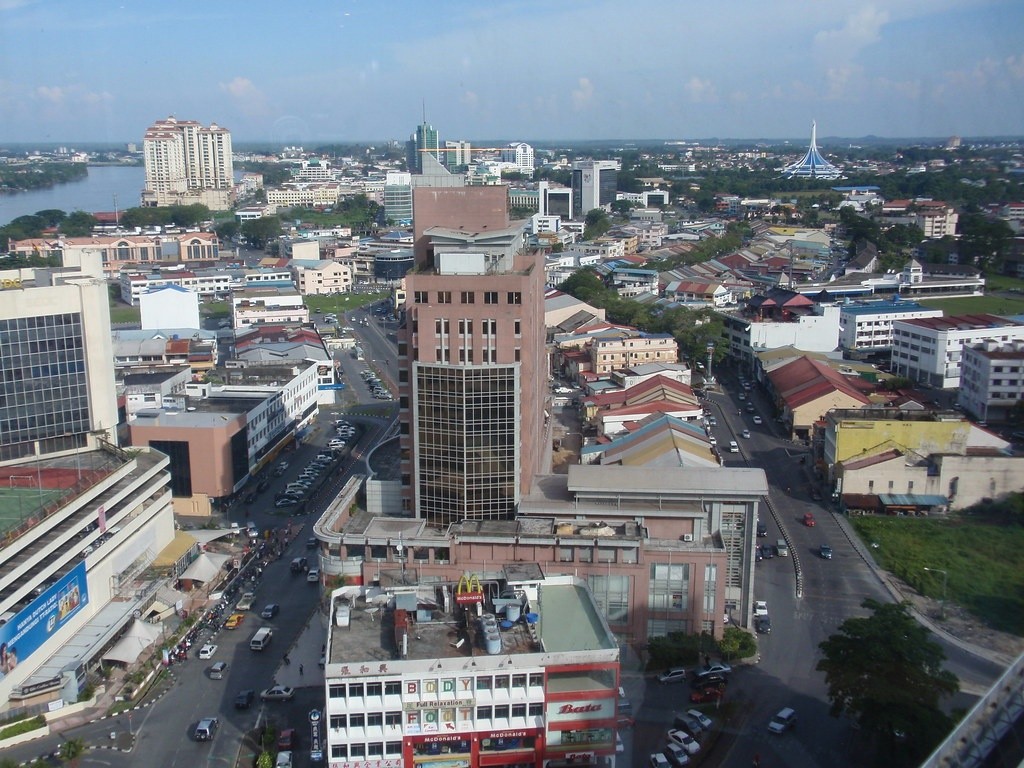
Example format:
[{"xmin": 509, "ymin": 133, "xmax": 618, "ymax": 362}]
[
  {"xmin": 235, "ymin": 689, "xmax": 254, "ymax": 709},
  {"xmin": 209, "ymin": 661, "xmax": 231, "ymax": 679},
  {"xmin": 768, "ymin": 707, "xmax": 797, "ymax": 734},
  {"xmin": 224, "ymin": 613, "xmax": 244, "ymax": 630},
  {"xmin": 197, "ymin": 644, "xmax": 217, "ymax": 660},
  {"xmin": 275, "ymin": 749, "xmax": 293, "ymax": 768},
  {"xmin": 174, "ymin": 306, "xmax": 839, "ymax": 768},
  {"xmin": 277, "ymin": 727, "xmax": 295, "ymax": 749},
  {"xmin": 259, "ymin": 684, "xmax": 297, "ymax": 703}
]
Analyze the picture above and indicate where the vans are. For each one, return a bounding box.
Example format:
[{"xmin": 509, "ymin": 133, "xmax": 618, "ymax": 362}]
[{"xmin": 249, "ymin": 627, "xmax": 273, "ymax": 651}]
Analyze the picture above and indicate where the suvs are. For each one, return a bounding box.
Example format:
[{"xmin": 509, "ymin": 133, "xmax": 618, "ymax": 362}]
[{"xmin": 193, "ymin": 717, "xmax": 219, "ymax": 741}]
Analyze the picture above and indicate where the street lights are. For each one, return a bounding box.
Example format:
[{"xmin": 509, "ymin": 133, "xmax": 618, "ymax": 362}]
[{"xmin": 923, "ymin": 567, "xmax": 947, "ymax": 621}]
